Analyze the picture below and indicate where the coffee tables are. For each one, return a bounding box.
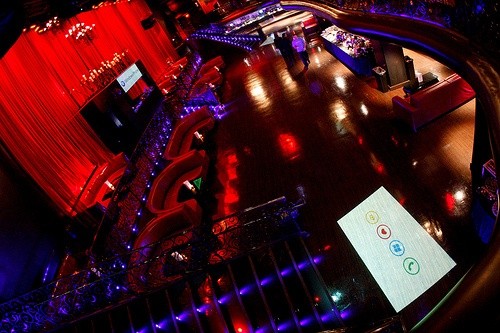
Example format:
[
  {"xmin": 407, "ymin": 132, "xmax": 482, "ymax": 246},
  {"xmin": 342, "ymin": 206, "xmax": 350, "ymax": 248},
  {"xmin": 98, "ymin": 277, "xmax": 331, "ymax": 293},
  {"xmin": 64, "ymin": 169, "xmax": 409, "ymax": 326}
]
[{"xmin": 403, "ymin": 72, "xmax": 441, "ymax": 96}]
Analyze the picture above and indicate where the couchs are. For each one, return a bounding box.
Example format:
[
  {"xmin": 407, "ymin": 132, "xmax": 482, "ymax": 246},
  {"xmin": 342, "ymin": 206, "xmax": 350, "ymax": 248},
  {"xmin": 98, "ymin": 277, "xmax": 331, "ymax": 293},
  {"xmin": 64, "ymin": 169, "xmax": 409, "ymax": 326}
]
[
  {"xmin": 163, "ymin": 108, "xmax": 217, "ymax": 165},
  {"xmin": 392, "ymin": 72, "xmax": 476, "ymax": 132},
  {"xmin": 126, "ymin": 207, "xmax": 200, "ymax": 297},
  {"xmin": 143, "ymin": 151, "xmax": 211, "ymax": 219}
]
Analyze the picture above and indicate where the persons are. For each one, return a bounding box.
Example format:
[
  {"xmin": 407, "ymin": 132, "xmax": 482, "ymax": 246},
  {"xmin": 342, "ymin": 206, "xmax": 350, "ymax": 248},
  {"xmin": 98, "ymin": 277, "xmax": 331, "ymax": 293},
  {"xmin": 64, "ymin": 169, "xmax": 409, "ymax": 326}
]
[
  {"xmin": 403, "ymin": 78, "xmax": 419, "ymax": 95},
  {"xmin": 274, "ymin": 30, "xmax": 311, "ymax": 70},
  {"xmin": 336, "ymin": 30, "xmax": 374, "ymax": 58}
]
